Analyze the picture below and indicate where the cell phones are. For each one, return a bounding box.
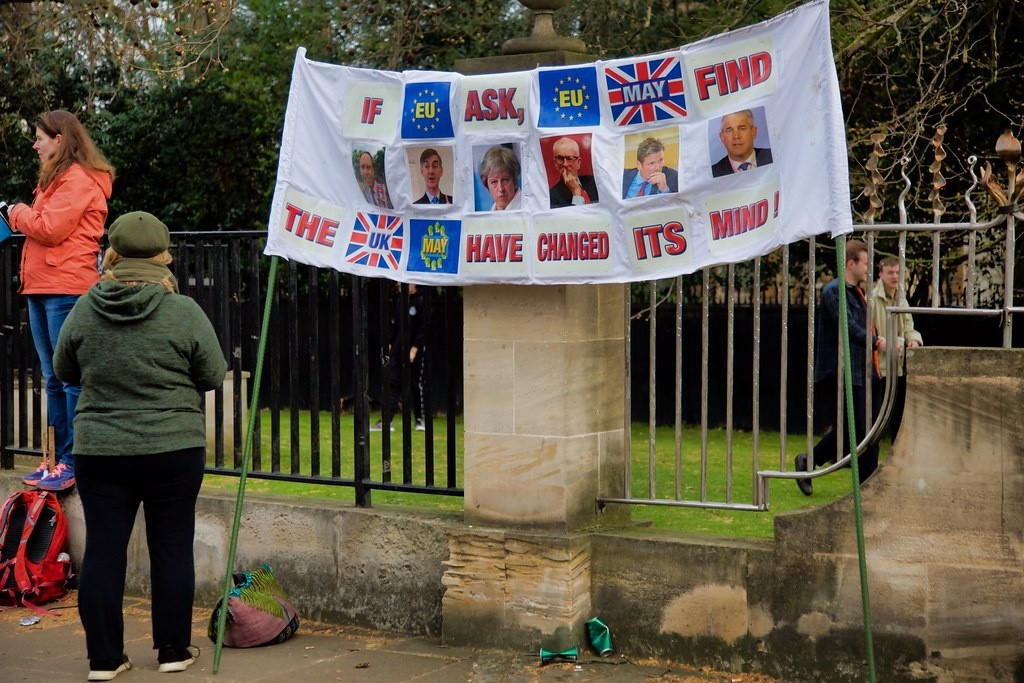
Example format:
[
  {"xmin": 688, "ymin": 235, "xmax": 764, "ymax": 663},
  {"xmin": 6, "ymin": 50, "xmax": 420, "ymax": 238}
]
[{"xmin": 0, "ymin": 201, "xmax": 17, "ymax": 233}]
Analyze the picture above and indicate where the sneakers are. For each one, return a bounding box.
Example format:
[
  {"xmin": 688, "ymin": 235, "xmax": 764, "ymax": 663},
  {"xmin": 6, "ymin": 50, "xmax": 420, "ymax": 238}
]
[
  {"xmin": 21, "ymin": 456, "xmax": 59, "ymax": 486},
  {"xmin": 157, "ymin": 644, "xmax": 200, "ymax": 672},
  {"xmin": 87, "ymin": 654, "xmax": 132, "ymax": 680},
  {"xmin": 36, "ymin": 462, "xmax": 75, "ymax": 491}
]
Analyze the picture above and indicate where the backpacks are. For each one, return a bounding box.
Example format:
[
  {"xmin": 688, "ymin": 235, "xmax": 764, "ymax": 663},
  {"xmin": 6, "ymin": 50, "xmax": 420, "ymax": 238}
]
[{"xmin": 0, "ymin": 489, "xmax": 76, "ymax": 616}]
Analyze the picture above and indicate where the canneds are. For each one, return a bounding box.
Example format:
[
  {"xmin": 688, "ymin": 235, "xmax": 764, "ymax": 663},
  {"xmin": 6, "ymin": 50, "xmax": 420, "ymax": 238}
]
[
  {"xmin": 585, "ymin": 617, "xmax": 614, "ymax": 658},
  {"xmin": 539, "ymin": 646, "xmax": 578, "ymax": 666}
]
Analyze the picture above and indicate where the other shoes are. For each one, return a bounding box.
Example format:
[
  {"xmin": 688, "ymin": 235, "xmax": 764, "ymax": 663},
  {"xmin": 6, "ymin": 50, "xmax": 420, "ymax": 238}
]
[{"xmin": 794, "ymin": 453, "xmax": 817, "ymax": 496}]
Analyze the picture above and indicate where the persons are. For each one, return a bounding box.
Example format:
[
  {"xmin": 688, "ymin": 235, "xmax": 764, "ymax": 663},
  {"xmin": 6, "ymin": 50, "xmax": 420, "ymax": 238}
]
[
  {"xmin": 864, "ymin": 257, "xmax": 924, "ymax": 445},
  {"xmin": 7, "ymin": 110, "xmax": 117, "ymax": 491},
  {"xmin": 358, "ymin": 152, "xmax": 393, "ymax": 210},
  {"xmin": 414, "ymin": 149, "xmax": 453, "ymax": 205},
  {"xmin": 623, "ymin": 138, "xmax": 678, "ymax": 199},
  {"xmin": 550, "ymin": 138, "xmax": 598, "ymax": 208},
  {"xmin": 369, "ymin": 282, "xmax": 426, "ymax": 432},
  {"xmin": 712, "ymin": 109, "xmax": 773, "ymax": 178},
  {"xmin": 51, "ymin": 210, "xmax": 229, "ymax": 682},
  {"xmin": 794, "ymin": 239, "xmax": 902, "ymax": 497},
  {"xmin": 479, "ymin": 146, "xmax": 522, "ymax": 211}
]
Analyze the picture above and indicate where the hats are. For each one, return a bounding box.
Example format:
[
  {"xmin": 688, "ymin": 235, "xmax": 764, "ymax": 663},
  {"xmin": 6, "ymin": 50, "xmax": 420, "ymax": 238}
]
[{"xmin": 107, "ymin": 211, "xmax": 170, "ymax": 258}]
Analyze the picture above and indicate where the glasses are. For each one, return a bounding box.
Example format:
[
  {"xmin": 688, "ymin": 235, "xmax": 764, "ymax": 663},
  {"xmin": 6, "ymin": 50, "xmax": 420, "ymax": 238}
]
[
  {"xmin": 555, "ymin": 155, "xmax": 578, "ymax": 163},
  {"xmin": 32, "ymin": 116, "xmax": 41, "ymax": 122}
]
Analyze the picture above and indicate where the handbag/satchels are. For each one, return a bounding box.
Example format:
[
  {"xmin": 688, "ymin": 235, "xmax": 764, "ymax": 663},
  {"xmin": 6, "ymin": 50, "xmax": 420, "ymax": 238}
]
[{"xmin": 207, "ymin": 565, "xmax": 300, "ymax": 647}]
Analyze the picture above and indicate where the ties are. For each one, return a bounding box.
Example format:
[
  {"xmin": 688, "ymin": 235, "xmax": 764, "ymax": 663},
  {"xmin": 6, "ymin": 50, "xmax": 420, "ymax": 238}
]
[
  {"xmin": 431, "ymin": 195, "xmax": 438, "ymax": 204},
  {"xmin": 856, "ymin": 286, "xmax": 883, "ymax": 380},
  {"xmin": 369, "ymin": 189, "xmax": 378, "ymax": 206},
  {"xmin": 739, "ymin": 162, "xmax": 749, "ymax": 172},
  {"xmin": 578, "ymin": 184, "xmax": 591, "ymax": 203},
  {"xmin": 637, "ymin": 181, "xmax": 647, "ymax": 197}
]
